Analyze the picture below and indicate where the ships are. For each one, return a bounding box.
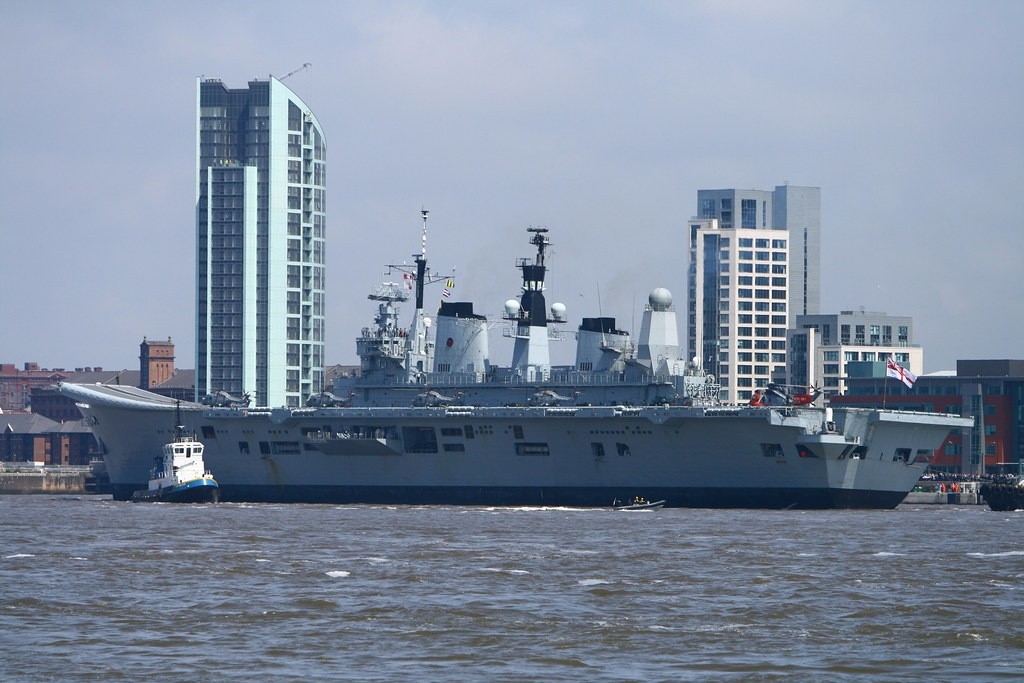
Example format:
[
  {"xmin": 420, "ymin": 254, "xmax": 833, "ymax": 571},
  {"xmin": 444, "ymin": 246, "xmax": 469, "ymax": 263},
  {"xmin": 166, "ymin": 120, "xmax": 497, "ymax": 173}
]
[{"xmin": 56, "ymin": 205, "xmax": 976, "ymax": 512}]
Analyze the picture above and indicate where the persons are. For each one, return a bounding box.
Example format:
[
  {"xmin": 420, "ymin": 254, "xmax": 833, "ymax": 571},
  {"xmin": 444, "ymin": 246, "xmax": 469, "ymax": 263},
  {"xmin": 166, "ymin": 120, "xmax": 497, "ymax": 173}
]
[
  {"xmin": 612, "ymin": 496, "xmax": 645, "ymax": 507},
  {"xmin": 361, "ymin": 322, "xmax": 409, "ymax": 337},
  {"xmin": 115, "ymin": 374, "xmax": 120, "ymax": 385},
  {"xmin": 919, "ymin": 471, "xmax": 1017, "ymax": 492}
]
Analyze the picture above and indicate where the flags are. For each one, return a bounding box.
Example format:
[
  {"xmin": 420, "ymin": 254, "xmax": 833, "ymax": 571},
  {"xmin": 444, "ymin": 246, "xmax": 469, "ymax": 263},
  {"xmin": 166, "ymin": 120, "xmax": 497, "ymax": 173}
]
[
  {"xmin": 443, "ymin": 280, "xmax": 455, "ymax": 298},
  {"xmin": 404, "ymin": 273, "xmax": 409, "ymax": 279},
  {"xmin": 885, "ymin": 357, "xmax": 918, "ymax": 389}
]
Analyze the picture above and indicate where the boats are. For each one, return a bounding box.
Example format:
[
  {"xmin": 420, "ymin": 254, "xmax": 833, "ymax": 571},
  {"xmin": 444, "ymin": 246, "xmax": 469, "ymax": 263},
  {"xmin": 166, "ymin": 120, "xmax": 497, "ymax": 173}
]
[{"xmin": 131, "ymin": 396, "xmax": 219, "ymax": 506}]
